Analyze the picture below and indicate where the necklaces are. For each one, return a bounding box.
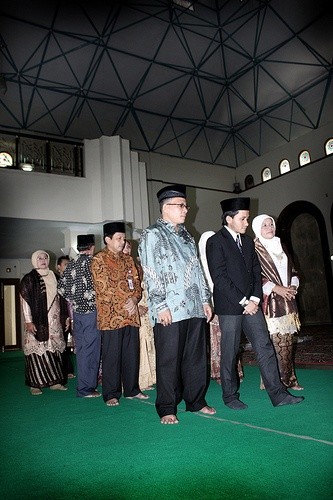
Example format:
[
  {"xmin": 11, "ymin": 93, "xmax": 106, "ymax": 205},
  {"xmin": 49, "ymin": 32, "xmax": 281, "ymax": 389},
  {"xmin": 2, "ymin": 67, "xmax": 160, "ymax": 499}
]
[{"xmin": 42, "ymin": 273, "xmax": 49, "ymax": 277}]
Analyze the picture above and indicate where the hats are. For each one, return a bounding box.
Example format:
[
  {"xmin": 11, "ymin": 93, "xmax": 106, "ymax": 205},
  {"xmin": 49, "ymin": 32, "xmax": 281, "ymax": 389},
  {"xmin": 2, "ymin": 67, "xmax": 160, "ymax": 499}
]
[
  {"xmin": 220, "ymin": 197, "xmax": 250, "ymax": 212},
  {"xmin": 156, "ymin": 184, "xmax": 186, "ymax": 202},
  {"xmin": 77, "ymin": 234, "xmax": 95, "ymax": 245},
  {"xmin": 103, "ymin": 222, "xmax": 125, "ymax": 234}
]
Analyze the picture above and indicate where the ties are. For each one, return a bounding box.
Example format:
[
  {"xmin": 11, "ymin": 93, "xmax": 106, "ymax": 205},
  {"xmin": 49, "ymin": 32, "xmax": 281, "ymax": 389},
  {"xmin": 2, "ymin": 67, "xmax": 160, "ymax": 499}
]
[{"xmin": 236, "ymin": 235, "xmax": 243, "ymax": 255}]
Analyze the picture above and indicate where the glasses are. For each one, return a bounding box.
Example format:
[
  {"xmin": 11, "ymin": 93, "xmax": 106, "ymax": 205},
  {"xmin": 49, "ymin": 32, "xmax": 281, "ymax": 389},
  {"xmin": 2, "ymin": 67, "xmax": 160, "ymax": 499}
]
[{"xmin": 167, "ymin": 204, "xmax": 191, "ymax": 210}]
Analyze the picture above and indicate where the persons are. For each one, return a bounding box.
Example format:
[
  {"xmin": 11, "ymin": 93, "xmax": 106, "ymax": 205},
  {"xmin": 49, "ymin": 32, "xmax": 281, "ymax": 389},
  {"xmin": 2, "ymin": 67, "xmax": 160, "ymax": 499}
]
[
  {"xmin": 18, "ymin": 250, "xmax": 69, "ymax": 395},
  {"xmin": 251, "ymin": 214, "xmax": 304, "ymax": 391},
  {"xmin": 90, "ymin": 222, "xmax": 150, "ymax": 406},
  {"xmin": 137, "ymin": 185, "xmax": 217, "ymax": 425},
  {"xmin": 206, "ymin": 197, "xmax": 305, "ymax": 411},
  {"xmin": 57, "ymin": 234, "xmax": 102, "ymax": 398},
  {"xmin": 196, "ymin": 230, "xmax": 244, "ymax": 383},
  {"xmin": 54, "ymin": 239, "xmax": 157, "ymax": 392}
]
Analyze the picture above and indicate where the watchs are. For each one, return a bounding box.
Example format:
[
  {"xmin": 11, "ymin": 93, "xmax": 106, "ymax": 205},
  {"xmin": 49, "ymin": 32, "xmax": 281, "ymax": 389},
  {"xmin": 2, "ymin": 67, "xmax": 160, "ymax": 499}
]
[{"xmin": 242, "ymin": 299, "xmax": 249, "ymax": 307}]
